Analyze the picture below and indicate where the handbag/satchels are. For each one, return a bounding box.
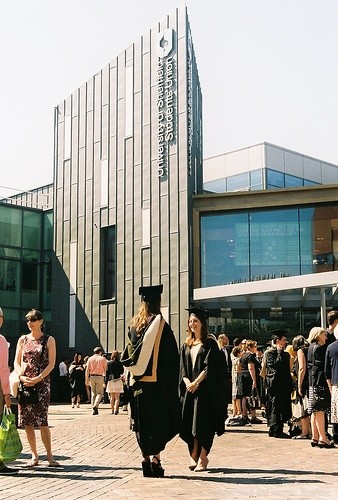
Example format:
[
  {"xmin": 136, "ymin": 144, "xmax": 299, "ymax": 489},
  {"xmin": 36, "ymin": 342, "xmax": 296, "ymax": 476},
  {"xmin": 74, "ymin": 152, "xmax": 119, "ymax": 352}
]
[
  {"xmin": 19, "ymin": 386, "xmax": 39, "ymax": 404},
  {"xmin": 0, "ymin": 404, "xmax": 22, "ymax": 462},
  {"xmin": 312, "ymin": 395, "xmax": 331, "ymax": 409},
  {"xmin": 247, "ymin": 386, "xmax": 261, "ymax": 408}
]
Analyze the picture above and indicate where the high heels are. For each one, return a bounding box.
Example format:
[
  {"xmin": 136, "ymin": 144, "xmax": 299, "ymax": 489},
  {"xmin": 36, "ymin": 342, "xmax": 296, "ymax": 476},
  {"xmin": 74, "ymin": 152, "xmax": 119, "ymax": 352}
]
[
  {"xmin": 142, "ymin": 455, "xmax": 164, "ymax": 477},
  {"xmin": 189, "ymin": 454, "xmax": 209, "ymax": 470}
]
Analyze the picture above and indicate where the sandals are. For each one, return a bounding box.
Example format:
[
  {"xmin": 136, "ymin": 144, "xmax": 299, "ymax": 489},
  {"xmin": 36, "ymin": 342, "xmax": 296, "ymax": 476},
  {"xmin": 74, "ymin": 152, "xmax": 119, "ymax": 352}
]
[
  {"xmin": 310, "ymin": 437, "xmax": 318, "ymax": 446},
  {"xmin": 318, "ymin": 440, "xmax": 334, "ymax": 447}
]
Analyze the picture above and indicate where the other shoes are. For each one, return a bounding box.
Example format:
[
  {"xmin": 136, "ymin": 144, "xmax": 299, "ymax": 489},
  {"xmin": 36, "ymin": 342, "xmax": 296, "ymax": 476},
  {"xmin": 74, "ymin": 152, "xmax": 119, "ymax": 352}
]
[
  {"xmin": 0, "ymin": 467, "xmax": 19, "ymax": 474},
  {"xmin": 76, "ymin": 402, "xmax": 80, "ymax": 408},
  {"xmin": 245, "ymin": 416, "xmax": 251, "ymax": 422},
  {"xmin": 251, "ymin": 416, "xmax": 262, "ymax": 423},
  {"xmin": 47, "ymin": 459, "xmax": 58, "ymax": 466},
  {"xmin": 93, "ymin": 406, "xmax": 98, "ymax": 415},
  {"xmin": 270, "ymin": 431, "xmax": 290, "ymax": 438},
  {"xmin": 71, "ymin": 402, "xmax": 74, "ymax": 408},
  {"xmin": 26, "ymin": 457, "xmax": 39, "ymax": 466},
  {"xmin": 292, "ymin": 434, "xmax": 312, "ymax": 438}
]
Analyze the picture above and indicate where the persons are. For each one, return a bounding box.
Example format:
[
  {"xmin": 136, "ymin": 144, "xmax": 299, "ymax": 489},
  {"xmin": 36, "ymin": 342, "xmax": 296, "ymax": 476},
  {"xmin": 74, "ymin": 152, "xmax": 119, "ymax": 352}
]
[
  {"xmin": 59, "ymin": 354, "xmax": 110, "ymax": 404},
  {"xmin": 0, "ymin": 306, "xmax": 20, "ymax": 474},
  {"xmin": 178, "ymin": 306, "xmax": 228, "ymax": 472},
  {"xmin": 218, "ymin": 311, "xmax": 338, "ymax": 449},
  {"xmin": 69, "ymin": 351, "xmax": 85, "ymax": 408},
  {"xmin": 120, "ymin": 284, "xmax": 183, "ymax": 477},
  {"xmin": 14, "ymin": 309, "xmax": 60, "ymax": 467},
  {"xmin": 86, "ymin": 347, "xmax": 109, "ymax": 414},
  {"xmin": 105, "ymin": 350, "xmax": 124, "ymax": 415}
]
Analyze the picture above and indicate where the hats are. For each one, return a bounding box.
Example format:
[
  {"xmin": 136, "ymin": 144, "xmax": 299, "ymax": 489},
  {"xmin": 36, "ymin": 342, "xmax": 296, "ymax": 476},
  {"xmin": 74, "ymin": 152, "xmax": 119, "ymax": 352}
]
[
  {"xmin": 185, "ymin": 307, "xmax": 211, "ymax": 321},
  {"xmin": 75, "ymin": 351, "xmax": 82, "ymax": 355},
  {"xmin": 268, "ymin": 329, "xmax": 288, "ymax": 337},
  {"xmin": 138, "ymin": 284, "xmax": 163, "ymax": 296}
]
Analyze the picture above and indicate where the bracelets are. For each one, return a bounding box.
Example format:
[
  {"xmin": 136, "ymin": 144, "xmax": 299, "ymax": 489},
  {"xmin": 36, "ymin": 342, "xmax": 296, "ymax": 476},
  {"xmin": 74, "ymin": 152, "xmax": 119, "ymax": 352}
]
[{"xmin": 39, "ymin": 373, "xmax": 43, "ymax": 381}]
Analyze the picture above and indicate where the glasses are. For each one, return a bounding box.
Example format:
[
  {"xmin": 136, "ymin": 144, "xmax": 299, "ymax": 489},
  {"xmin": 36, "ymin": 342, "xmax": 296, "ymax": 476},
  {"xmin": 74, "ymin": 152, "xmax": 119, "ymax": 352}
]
[
  {"xmin": 26, "ymin": 318, "xmax": 41, "ymax": 322},
  {"xmin": 252, "ymin": 346, "xmax": 256, "ymax": 348}
]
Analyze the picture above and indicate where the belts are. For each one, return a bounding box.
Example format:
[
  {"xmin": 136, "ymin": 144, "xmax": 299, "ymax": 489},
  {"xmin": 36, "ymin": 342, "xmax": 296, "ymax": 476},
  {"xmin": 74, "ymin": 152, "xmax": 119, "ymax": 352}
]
[{"xmin": 91, "ymin": 374, "xmax": 102, "ymax": 376}]
